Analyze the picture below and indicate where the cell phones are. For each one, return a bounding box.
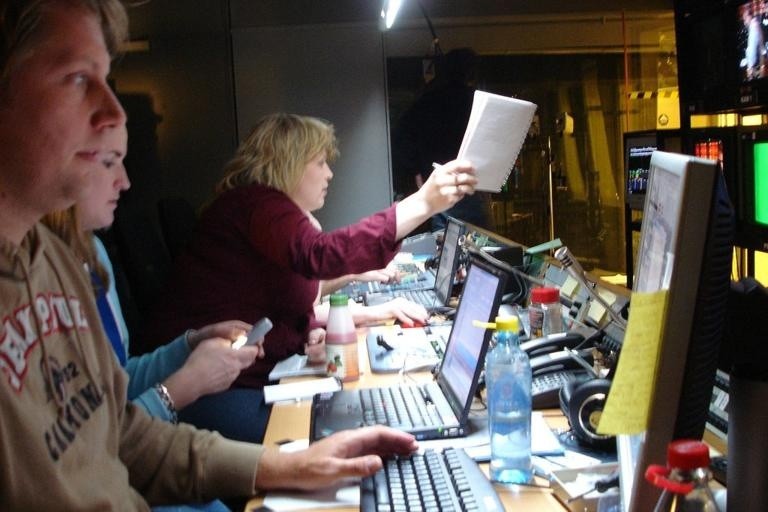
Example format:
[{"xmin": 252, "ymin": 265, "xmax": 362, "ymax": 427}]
[{"xmin": 232, "ymin": 317, "xmax": 274, "ymax": 346}]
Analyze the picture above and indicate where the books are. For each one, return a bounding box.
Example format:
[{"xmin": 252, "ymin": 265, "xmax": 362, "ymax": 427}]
[{"xmin": 456, "ymin": 90, "xmax": 538, "ymax": 194}]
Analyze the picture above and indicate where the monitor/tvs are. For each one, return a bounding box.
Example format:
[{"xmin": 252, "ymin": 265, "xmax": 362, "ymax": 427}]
[
  {"xmin": 624, "ymin": 0, "xmax": 768, "ymax": 254},
  {"xmin": 615, "ymin": 150, "xmax": 717, "ymax": 512}
]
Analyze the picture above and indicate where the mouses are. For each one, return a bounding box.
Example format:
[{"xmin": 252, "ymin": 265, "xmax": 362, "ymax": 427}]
[{"xmin": 393, "ymin": 317, "xmax": 428, "ymax": 328}]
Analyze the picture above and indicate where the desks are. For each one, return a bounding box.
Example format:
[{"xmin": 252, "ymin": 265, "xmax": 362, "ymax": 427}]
[{"xmin": 245, "ymin": 218, "xmax": 727, "ymax": 511}]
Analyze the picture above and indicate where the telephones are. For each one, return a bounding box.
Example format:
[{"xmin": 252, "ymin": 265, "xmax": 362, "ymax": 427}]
[{"xmin": 520, "ymin": 332, "xmax": 598, "ymax": 410}]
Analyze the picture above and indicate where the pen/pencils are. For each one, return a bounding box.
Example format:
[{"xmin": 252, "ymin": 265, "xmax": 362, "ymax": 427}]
[{"xmin": 431, "ymin": 162, "xmax": 457, "ymax": 175}]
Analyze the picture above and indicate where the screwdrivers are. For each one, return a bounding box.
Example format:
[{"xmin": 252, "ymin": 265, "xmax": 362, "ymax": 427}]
[{"xmin": 566, "ymin": 471, "xmax": 619, "ymax": 505}]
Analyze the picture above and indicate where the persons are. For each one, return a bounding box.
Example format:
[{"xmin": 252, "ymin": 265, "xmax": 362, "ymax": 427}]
[
  {"xmin": 142, "ymin": 111, "xmax": 481, "ymax": 445},
  {"xmin": 740, "ymin": 5, "xmax": 764, "ymax": 81},
  {"xmin": 760, "ymin": 0, "xmax": 768, "ymax": 55},
  {"xmin": 0, "ymin": 0, "xmax": 419, "ymax": 512},
  {"xmin": 391, "ymin": 46, "xmax": 495, "ymax": 233},
  {"xmin": 38, "ymin": 122, "xmax": 268, "ymax": 512}
]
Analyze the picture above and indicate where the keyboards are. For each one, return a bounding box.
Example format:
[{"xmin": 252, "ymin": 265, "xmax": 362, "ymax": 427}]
[{"xmin": 360, "ymin": 447, "xmax": 507, "ymax": 512}]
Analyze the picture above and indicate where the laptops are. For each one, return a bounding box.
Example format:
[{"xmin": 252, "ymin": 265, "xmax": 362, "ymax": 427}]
[
  {"xmin": 308, "ymin": 259, "xmax": 509, "ymax": 446},
  {"xmin": 362, "ymin": 215, "xmax": 466, "ymax": 313}
]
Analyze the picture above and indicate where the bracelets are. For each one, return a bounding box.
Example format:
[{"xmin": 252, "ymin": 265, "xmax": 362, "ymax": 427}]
[{"xmin": 155, "ymin": 382, "xmax": 178, "ymax": 423}]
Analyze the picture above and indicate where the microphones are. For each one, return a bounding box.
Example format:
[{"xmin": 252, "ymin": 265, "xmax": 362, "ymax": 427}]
[{"xmin": 553, "ymin": 246, "xmax": 627, "ymax": 330}]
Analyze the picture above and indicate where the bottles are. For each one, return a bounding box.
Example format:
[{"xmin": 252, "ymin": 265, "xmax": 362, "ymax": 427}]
[
  {"xmin": 325, "ymin": 294, "xmax": 360, "ymax": 383},
  {"xmin": 484, "ymin": 314, "xmax": 533, "ymax": 486},
  {"xmin": 653, "ymin": 439, "xmax": 721, "ymax": 512},
  {"xmin": 527, "ymin": 287, "xmax": 565, "ymax": 341}
]
